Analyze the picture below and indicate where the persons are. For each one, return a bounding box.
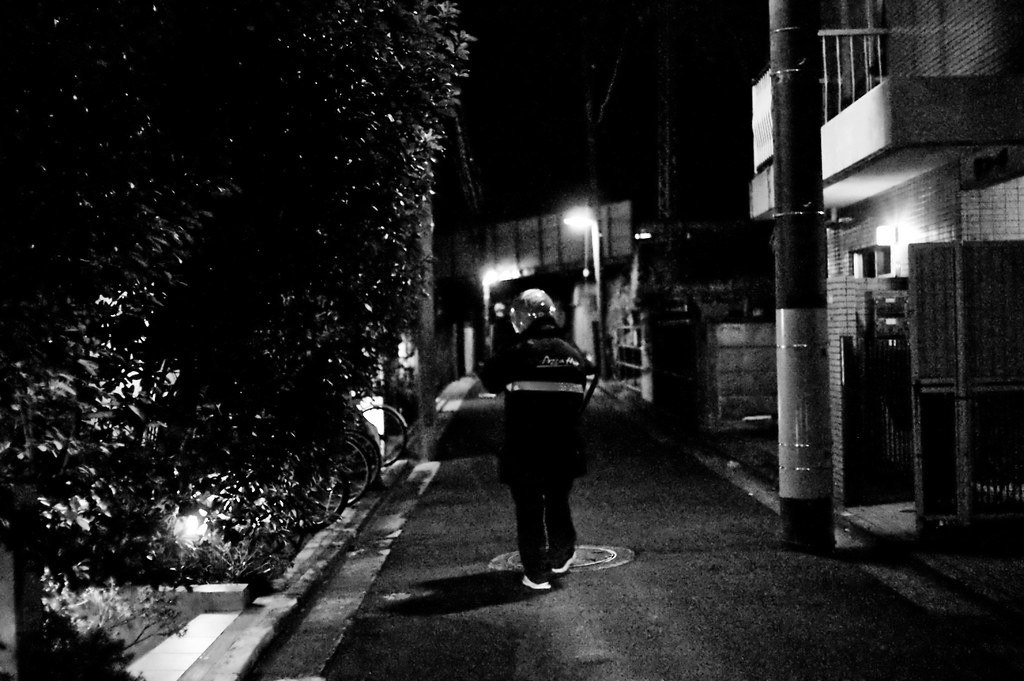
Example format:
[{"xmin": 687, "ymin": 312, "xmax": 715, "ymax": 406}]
[{"xmin": 478, "ymin": 287, "xmax": 588, "ymax": 589}]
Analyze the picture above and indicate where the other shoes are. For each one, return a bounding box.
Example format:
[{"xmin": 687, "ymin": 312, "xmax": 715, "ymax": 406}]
[
  {"xmin": 551, "ymin": 547, "xmax": 578, "ymax": 574},
  {"xmin": 522, "ymin": 571, "xmax": 551, "ymax": 591}
]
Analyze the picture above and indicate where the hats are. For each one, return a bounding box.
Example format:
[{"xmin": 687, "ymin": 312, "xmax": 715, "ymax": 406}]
[{"xmin": 513, "ymin": 288, "xmax": 552, "ymax": 312}]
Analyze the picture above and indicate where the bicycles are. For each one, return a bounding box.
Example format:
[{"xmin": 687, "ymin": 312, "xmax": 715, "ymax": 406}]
[{"xmin": 286, "ymin": 399, "xmax": 410, "ymax": 534}]
